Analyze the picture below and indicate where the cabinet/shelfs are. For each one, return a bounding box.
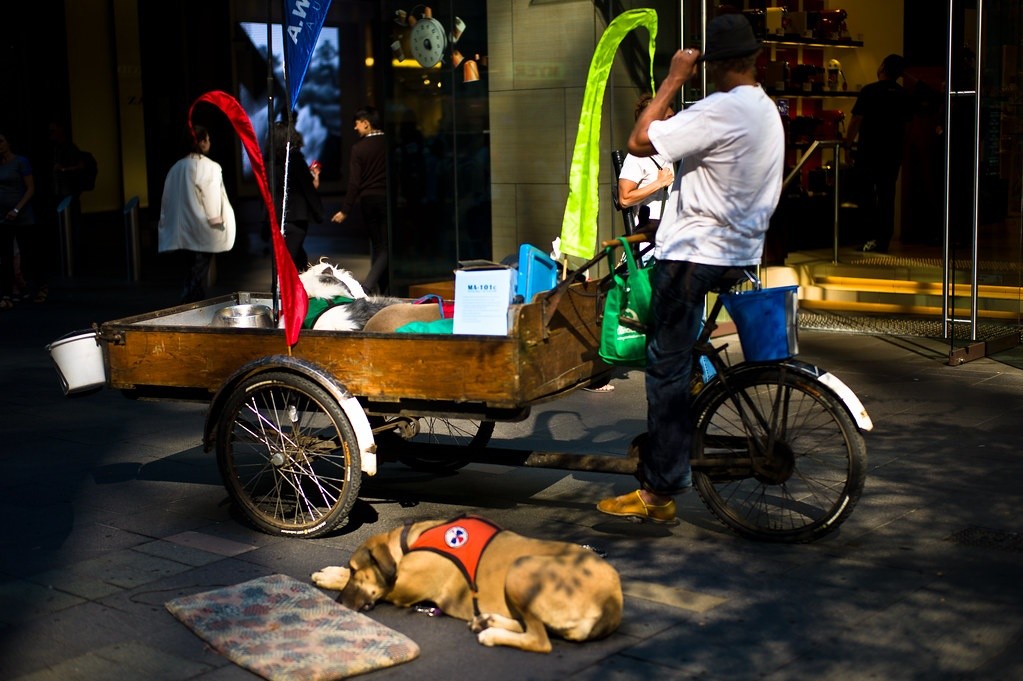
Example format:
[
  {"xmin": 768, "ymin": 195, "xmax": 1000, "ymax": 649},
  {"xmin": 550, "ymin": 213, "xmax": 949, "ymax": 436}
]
[{"xmin": 691, "ymin": 36, "xmax": 864, "ymax": 197}]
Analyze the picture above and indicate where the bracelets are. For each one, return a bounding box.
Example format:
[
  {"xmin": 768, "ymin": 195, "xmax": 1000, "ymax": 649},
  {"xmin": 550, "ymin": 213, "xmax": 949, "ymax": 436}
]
[{"xmin": 14, "ymin": 208, "xmax": 19, "ymax": 213}]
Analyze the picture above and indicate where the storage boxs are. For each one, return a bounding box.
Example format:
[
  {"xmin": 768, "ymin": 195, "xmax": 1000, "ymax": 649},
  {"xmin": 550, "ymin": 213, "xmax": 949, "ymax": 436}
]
[{"xmin": 453, "ymin": 261, "xmax": 518, "ymax": 335}]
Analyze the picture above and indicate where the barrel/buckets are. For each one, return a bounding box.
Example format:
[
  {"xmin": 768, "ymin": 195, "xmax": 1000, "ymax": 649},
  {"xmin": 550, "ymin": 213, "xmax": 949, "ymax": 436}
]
[
  {"xmin": 214, "ymin": 304, "xmax": 273, "ymax": 328},
  {"xmin": 717, "ymin": 285, "xmax": 800, "ymax": 363},
  {"xmin": 44, "ymin": 332, "xmax": 108, "ymax": 395}
]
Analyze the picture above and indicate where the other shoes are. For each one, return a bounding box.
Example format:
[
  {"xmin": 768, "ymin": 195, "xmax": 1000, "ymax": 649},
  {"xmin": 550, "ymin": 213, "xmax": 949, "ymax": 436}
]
[
  {"xmin": 22, "ymin": 286, "xmax": 31, "ymax": 298},
  {"xmin": 582, "ymin": 384, "xmax": 615, "ymax": 393},
  {"xmin": 12, "ymin": 294, "xmax": 20, "ymax": 301},
  {"xmin": 34, "ymin": 287, "xmax": 48, "ymax": 302},
  {"xmin": 690, "ymin": 373, "xmax": 704, "ymax": 397},
  {"xmin": 862, "ymin": 240, "xmax": 878, "ymax": 252},
  {"xmin": 596, "ymin": 489, "xmax": 680, "ymax": 527}
]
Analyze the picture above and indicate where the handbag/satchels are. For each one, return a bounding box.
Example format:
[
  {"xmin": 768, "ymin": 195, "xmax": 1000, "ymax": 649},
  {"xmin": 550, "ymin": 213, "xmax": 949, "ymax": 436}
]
[{"xmin": 599, "ymin": 240, "xmax": 652, "ymax": 364}]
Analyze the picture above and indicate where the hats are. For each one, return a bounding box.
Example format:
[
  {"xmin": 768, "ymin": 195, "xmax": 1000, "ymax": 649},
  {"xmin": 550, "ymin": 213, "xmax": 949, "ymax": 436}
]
[{"xmin": 695, "ymin": 14, "xmax": 761, "ymax": 64}]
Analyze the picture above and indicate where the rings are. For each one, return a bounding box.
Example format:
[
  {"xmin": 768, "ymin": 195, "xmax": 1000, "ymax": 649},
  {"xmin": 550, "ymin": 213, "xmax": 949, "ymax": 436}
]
[{"xmin": 685, "ymin": 49, "xmax": 692, "ymax": 55}]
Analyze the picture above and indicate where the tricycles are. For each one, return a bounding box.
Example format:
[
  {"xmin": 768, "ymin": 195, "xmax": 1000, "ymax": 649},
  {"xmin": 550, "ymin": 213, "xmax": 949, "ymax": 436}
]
[{"xmin": 92, "ymin": 148, "xmax": 878, "ymax": 547}]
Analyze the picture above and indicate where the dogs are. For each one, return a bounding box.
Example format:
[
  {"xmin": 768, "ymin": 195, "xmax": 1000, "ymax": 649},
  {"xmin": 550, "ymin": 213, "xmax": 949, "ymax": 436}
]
[
  {"xmin": 274, "ymin": 257, "xmax": 455, "ymax": 334},
  {"xmin": 309, "ymin": 513, "xmax": 623, "ymax": 653}
]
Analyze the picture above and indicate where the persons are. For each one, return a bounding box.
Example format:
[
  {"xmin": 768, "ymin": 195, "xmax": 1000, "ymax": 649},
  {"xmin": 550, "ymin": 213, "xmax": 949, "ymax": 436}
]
[
  {"xmin": 598, "ymin": 15, "xmax": 785, "ymax": 522},
  {"xmin": 582, "ymin": 95, "xmax": 676, "ymax": 392},
  {"xmin": 845, "ymin": 53, "xmax": 912, "ymax": 251},
  {"xmin": 265, "ymin": 125, "xmax": 323, "ymax": 292},
  {"xmin": 158, "ymin": 126, "xmax": 236, "ymax": 303},
  {"xmin": 328, "ymin": 108, "xmax": 388, "ymax": 295},
  {"xmin": 0, "ymin": 121, "xmax": 84, "ymax": 309}
]
